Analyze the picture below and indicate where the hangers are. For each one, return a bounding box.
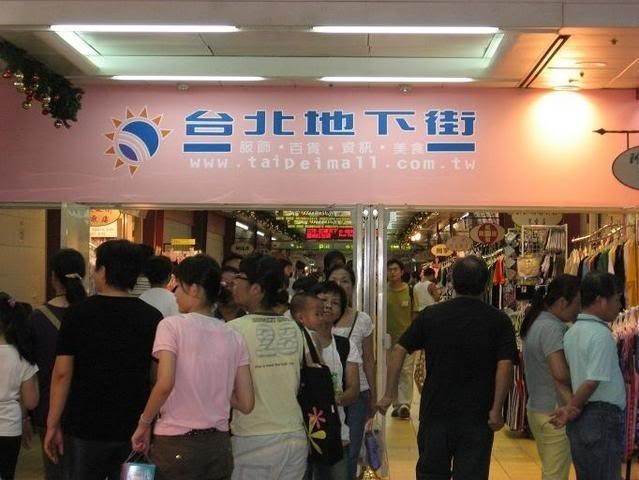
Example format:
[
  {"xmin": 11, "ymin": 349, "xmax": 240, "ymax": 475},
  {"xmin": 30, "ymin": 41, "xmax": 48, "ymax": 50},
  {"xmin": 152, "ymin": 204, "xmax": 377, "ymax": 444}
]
[{"xmin": 611, "ymin": 306, "xmax": 636, "ymax": 331}]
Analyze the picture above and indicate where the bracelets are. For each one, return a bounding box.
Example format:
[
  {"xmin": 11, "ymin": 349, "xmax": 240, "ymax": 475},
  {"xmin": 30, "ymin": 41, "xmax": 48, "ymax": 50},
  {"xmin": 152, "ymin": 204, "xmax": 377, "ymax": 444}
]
[
  {"xmin": 23, "ymin": 415, "xmax": 31, "ymax": 425},
  {"xmin": 137, "ymin": 412, "xmax": 151, "ymax": 425}
]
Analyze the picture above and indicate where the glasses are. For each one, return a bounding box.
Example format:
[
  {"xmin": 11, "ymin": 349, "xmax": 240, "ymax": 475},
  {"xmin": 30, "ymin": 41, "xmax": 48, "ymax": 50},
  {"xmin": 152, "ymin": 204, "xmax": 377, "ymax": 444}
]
[{"xmin": 233, "ymin": 273, "xmax": 248, "ymax": 285}]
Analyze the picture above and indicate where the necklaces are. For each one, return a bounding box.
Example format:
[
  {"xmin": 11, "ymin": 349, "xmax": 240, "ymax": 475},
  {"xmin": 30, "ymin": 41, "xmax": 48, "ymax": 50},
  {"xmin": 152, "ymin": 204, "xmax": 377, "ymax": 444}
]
[{"xmin": 55, "ymin": 293, "xmax": 67, "ymax": 297}]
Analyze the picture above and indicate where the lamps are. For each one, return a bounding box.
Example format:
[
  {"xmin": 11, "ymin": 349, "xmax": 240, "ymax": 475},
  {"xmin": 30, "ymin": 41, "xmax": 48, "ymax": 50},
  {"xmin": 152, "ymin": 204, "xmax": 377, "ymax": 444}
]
[{"xmin": 549, "ymin": 67, "xmax": 582, "ymax": 91}]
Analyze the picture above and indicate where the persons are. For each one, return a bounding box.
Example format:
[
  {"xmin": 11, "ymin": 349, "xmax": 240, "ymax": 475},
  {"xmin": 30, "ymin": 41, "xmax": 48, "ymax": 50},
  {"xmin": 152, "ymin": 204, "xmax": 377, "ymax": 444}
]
[
  {"xmin": 138, "ymin": 256, "xmax": 183, "ymax": 405},
  {"xmin": 374, "ymin": 255, "xmax": 519, "ymax": 480},
  {"xmin": 0, "ymin": 289, "xmax": 41, "ymax": 480},
  {"xmin": 546, "ymin": 271, "xmax": 628, "ymax": 480},
  {"xmin": 520, "ymin": 274, "xmax": 581, "ymax": 480},
  {"xmin": 18, "ymin": 248, "xmax": 92, "ymax": 480},
  {"xmin": 42, "ymin": 238, "xmax": 164, "ymax": 480},
  {"xmin": 134, "ymin": 253, "xmax": 254, "ymax": 480},
  {"xmin": 213, "ymin": 249, "xmax": 447, "ymax": 479},
  {"xmin": 129, "ymin": 244, "xmax": 154, "ymax": 294}
]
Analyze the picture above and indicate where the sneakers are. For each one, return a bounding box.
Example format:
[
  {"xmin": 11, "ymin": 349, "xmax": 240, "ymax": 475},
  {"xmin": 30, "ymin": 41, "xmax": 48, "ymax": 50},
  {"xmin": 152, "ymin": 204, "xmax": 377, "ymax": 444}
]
[
  {"xmin": 398, "ymin": 404, "xmax": 410, "ymax": 418},
  {"xmin": 391, "ymin": 405, "xmax": 400, "ymax": 416}
]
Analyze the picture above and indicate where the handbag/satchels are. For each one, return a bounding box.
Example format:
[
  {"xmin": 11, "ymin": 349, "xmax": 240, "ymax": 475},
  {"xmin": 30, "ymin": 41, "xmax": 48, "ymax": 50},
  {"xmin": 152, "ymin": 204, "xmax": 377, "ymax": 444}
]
[
  {"xmin": 355, "ymin": 430, "xmax": 390, "ymax": 480},
  {"xmin": 297, "ymin": 362, "xmax": 344, "ymax": 467},
  {"xmin": 121, "ymin": 461, "xmax": 157, "ymax": 480}
]
